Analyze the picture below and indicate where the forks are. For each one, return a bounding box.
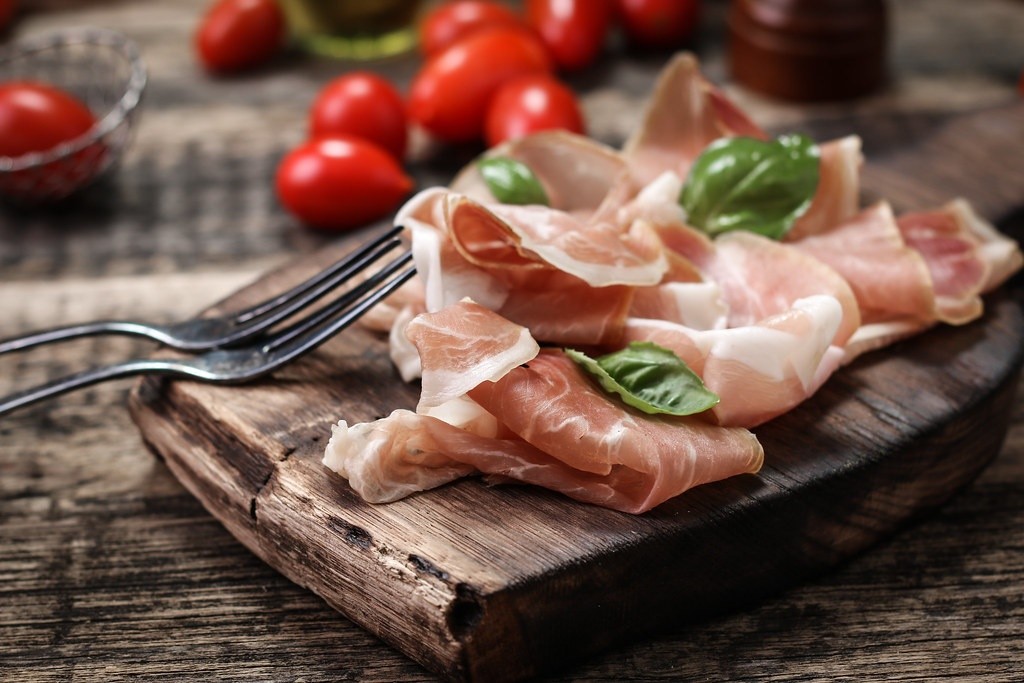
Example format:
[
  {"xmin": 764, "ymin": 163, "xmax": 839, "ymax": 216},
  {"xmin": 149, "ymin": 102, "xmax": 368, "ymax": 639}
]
[
  {"xmin": 1, "ymin": 224, "xmax": 405, "ymax": 366},
  {"xmin": 0, "ymin": 237, "xmax": 419, "ymax": 434}
]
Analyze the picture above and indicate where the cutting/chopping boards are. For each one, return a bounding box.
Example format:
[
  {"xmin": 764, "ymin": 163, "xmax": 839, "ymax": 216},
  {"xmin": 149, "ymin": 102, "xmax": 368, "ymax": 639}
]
[{"xmin": 117, "ymin": 130, "xmax": 1024, "ymax": 679}]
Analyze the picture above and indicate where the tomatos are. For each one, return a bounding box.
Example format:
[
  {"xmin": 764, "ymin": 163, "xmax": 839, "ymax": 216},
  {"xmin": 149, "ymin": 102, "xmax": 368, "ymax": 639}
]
[
  {"xmin": 0, "ymin": 80, "xmax": 106, "ymax": 198},
  {"xmin": 276, "ymin": 0, "xmax": 700, "ymax": 226},
  {"xmin": 200, "ymin": 0, "xmax": 274, "ymax": 72}
]
